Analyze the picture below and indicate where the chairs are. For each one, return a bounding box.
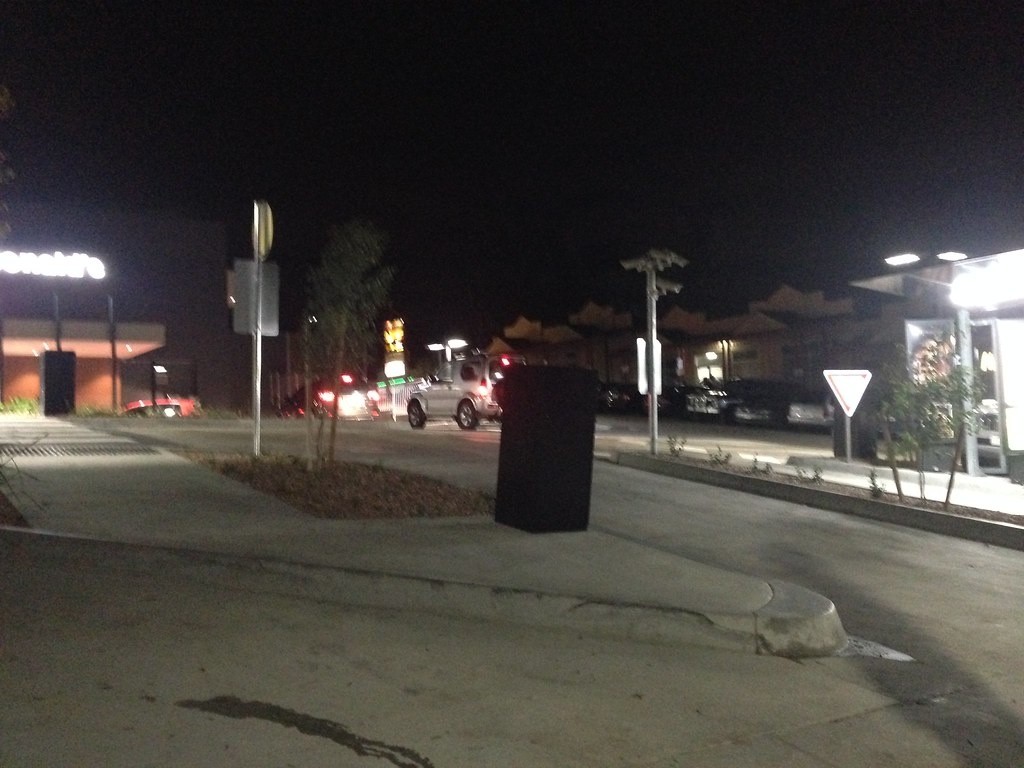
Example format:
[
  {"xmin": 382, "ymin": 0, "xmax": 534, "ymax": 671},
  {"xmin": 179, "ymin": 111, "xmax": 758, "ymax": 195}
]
[{"xmin": 461, "ymin": 367, "xmax": 476, "ymax": 381}]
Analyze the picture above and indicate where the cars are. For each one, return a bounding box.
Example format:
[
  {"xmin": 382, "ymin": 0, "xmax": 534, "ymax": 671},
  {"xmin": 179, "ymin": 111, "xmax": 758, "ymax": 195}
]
[
  {"xmin": 407, "ymin": 353, "xmax": 527, "ymax": 432},
  {"xmin": 276, "ymin": 375, "xmax": 381, "ymax": 421},
  {"xmin": 632, "ymin": 379, "xmax": 834, "ymax": 434},
  {"xmin": 598, "ymin": 383, "xmax": 631, "ymax": 415},
  {"xmin": 118, "ymin": 398, "xmax": 197, "ymax": 417}
]
